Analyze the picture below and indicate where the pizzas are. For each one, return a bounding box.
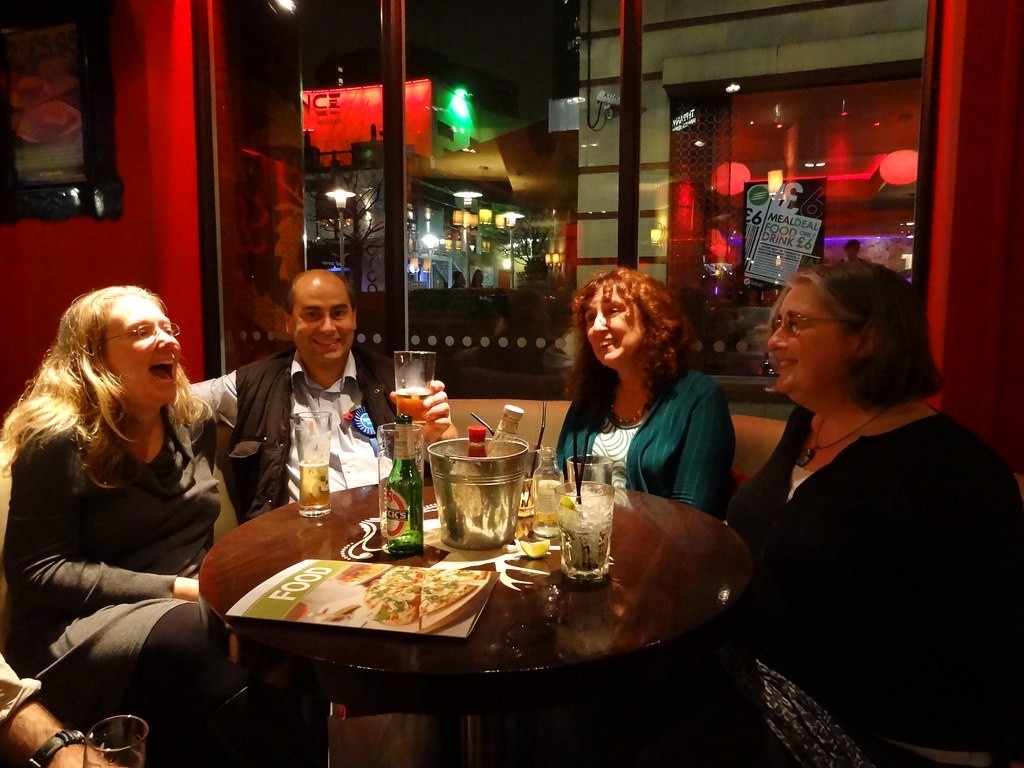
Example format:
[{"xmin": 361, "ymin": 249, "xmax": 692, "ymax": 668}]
[{"xmin": 363, "ymin": 565, "xmax": 488, "ymax": 624}]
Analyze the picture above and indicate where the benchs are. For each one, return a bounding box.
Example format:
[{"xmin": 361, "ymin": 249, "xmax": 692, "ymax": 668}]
[{"xmin": 0, "ymin": 399, "xmax": 789, "ymax": 662}]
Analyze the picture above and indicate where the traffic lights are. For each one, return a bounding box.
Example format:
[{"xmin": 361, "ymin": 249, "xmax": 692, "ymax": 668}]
[{"xmin": 351, "ymin": 140, "xmax": 385, "ymax": 171}]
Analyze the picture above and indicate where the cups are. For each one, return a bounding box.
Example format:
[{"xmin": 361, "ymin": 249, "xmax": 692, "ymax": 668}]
[
  {"xmin": 377, "ymin": 422, "xmax": 423, "ymax": 538},
  {"xmin": 555, "ymin": 482, "xmax": 614, "ymax": 580},
  {"xmin": 83, "ymin": 715, "xmax": 150, "ymax": 768},
  {"xmin": 292, "ymin": 411, "xmax": 333, "ymax": 517},
  {"xmin": 517, "ymin": 446, "xmax": 542, "ymax": 518},
  {"xmin": 393, "ymin": 351, "xmax": 437, "ymax": 426},
  {"xmin": 567, "ymin": 455, "xmax": 613, "ymax": 489}
]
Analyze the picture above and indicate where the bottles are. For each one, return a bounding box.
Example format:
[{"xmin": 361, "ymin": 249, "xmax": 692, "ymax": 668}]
[
  {"xmin": 385, "ymin": 414, "xmax": 423, "ymax": 556},
  {"xmin": 467, "ymin": 425, "xmax": 486, "ymax": 458},
  {"xmin": 487, "ymin": 404, "xmax": 523, "ymax": 459},
  {"xmin": 532, "ymin": 446, "xmax": 565, "ymax": 538}
]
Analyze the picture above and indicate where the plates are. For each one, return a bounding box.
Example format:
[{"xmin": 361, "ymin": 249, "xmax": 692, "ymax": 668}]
[{"xmin": 316, "ymin": 598, "xmax": 369, "ymax": 627}]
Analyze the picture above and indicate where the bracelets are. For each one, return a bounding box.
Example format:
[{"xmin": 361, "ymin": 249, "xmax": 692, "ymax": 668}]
[{"xmin": 27, "ymin": 730, "xmax": 87, "ymax": 768}]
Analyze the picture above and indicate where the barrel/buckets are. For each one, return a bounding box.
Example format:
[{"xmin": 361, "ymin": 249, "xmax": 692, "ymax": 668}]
[{"xmin": 427, "ymin": 437, "xmax": 528, "ymax": 551}]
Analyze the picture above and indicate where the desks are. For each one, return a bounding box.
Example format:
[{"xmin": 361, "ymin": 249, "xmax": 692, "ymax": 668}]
[{"xmin": 199, "ymin": 479, "xmax": 753, "ymax": 768}]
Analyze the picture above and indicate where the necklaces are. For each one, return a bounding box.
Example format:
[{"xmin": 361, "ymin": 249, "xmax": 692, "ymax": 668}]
[
  {"xmin": 611, "ymin": 405, "xmax": 643, "ymax": 424},
  {"xmin": 796, "ymin": 407, "xmax": 889, "ymax": 466}
]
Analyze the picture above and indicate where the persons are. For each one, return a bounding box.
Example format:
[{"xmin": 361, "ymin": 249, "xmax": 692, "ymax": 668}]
[
  {"xmin": 452, "ymin": 271, "xmax": 466, "ymax": 288},
  {"xmin": 3, "ymin": 285, "xmax": 321, "ymax": 768},
  {"xmin": 472, "ymin": 269, "xmax": 483, "ymax": 287},
  {"xmin": 541, "ymin": 262, "xmax": 1024, "ymax": 768},
  {"xmin": 553, "ymin": 266, "xmax": 736, "ymax": 518},
  {"xmin": 182, "ymin": 269, "xmax": 451, "ymax": 526},
  {"xmin": 0, "ymin": 652, "xmax": 121, "ymax": 768},
  {"xmin": 840, "ymin": 239, "xmax": 865, "ymax": 262}
]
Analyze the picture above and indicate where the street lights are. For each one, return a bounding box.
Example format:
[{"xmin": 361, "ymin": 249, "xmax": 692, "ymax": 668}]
[
  {"xmin": 452, "ymin": 186, "xmax": 484, "ymax": 287},
  {"xmin": 325, "ymin": 185, "xmax": 357, "ymax": 278},
  {"xmin": 500, "ymin": 210, "xmax": 526, "ymax": 289}
]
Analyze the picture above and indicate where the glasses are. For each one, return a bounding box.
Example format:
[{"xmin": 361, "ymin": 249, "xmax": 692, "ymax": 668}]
[
  {"xmin": 775, "ymin": 315, "xmax": 847, "ymax": 335},
  {"xmin": 101, "ymin": 323, "xmax": 180, "ymax": 342}
]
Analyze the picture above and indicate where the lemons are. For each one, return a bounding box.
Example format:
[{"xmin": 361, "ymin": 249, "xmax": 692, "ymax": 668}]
[
  {"xmin": 561, "ymin": 495, "xmax": 578, "ymax": 513},
  {"xmin": 520, "ymin": 539, "xmax": 550, "ymax": 558}
]
